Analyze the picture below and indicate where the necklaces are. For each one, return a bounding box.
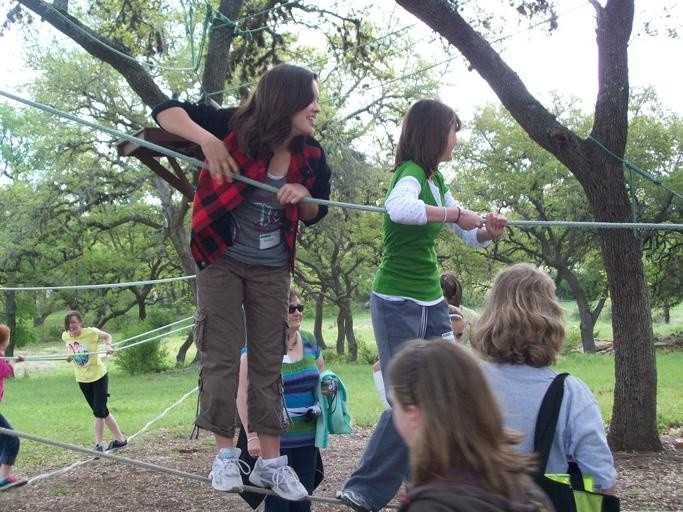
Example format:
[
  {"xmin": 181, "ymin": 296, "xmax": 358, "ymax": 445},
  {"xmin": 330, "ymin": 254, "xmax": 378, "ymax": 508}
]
[{"xmin": 286, "ymin": 334, "xmax": 300, "ymax": 351}]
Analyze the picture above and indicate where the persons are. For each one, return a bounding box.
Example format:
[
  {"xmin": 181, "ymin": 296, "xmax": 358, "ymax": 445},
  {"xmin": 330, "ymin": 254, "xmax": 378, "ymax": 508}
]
[
  {"xmin": 446, "ymin": 303, "xmax": 466, "ymax": 343},
  {"xmin": 152, "ymin": 65, "xmax": 332, "ymax": 503},
  {"xmin": 0, "ymin": 323, "xmax": 25, "ymax": 488},
  {"xmin": 467, "ymin": 263, "xmax": 617, "ymax": 495},
  {"xmin": 235, "ymin": 287, "xmax": 352, "ymax": 512},
  {"xmin": 61, "ymin": 313, "xmax": 128, "ymax": 454},
  {"xmin": 436, "ymin": 272, "xmax": 482, "ymax": 351},
  {"xmin": 337, "ymin": 97, "xmax": 507, "ymax": 512},
  {"xmin": 386, "ymin": 339, "xmax": 556, "ymax": 512}
]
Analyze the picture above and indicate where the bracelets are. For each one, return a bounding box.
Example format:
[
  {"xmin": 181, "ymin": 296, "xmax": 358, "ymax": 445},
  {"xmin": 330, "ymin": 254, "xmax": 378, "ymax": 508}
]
[
  {"xmin": 441, "ymin": 206, "xmax": 448, "ymax": 226},
  {"xmin": 455, "ymin": 206, "xmax": 462, "ymax": 225}
]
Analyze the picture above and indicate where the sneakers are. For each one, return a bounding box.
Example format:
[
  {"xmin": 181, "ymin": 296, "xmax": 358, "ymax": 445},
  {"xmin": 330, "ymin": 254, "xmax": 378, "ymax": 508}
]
[
  {"xmin": 208, "ymin": 448, "xmax": 251, "ymax": 493},
  {"xmin": 106, "ymin": 440, "xmax": 128, "ymax": 452},
  {"xmin": 336, "ymin": 489, "xmax": 372, "ymax": 512},
  {"xmin": 249, "ymin": 455, "xmax": 308, "ymax": 502},
  {"xmin": 95, "ymin": 443, "xmax": 105, "ymax": 461},
  {"xmin": 1, "ymin": 477, "xmax": 29, "ymax": 492}
]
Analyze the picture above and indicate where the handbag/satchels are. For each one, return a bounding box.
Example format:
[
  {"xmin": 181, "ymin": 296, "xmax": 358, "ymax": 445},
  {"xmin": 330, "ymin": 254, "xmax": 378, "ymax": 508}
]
[{"xmin": 527, "ymin": 372, "xmax": 621, "ymax": 508}]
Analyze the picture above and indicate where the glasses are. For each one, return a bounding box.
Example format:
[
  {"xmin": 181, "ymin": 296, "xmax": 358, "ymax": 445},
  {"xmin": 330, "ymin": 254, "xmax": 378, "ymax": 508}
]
[{"xmin": 289, "ymin": 304, "xmax": 304, "ymax": 315}]
